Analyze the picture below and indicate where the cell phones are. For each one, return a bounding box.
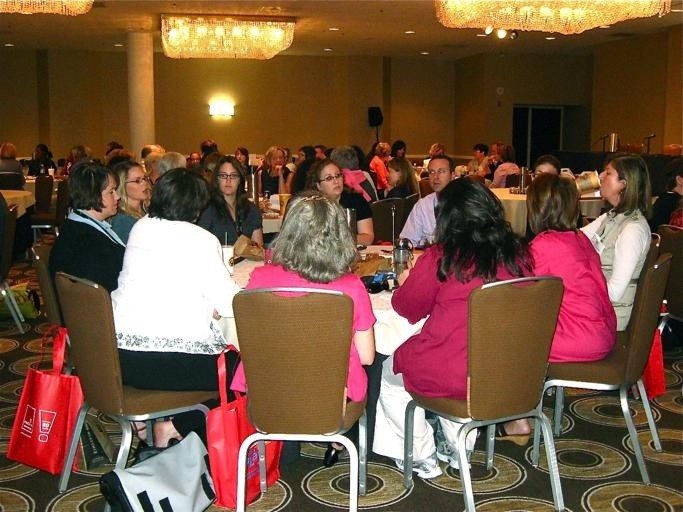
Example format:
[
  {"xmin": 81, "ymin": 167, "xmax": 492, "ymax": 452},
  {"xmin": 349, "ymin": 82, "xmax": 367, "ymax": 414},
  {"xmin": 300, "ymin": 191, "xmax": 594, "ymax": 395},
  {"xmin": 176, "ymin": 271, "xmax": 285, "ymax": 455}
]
[{"xmin": 372, "ymin": 269, "xmax": 388, "ymax": 285}]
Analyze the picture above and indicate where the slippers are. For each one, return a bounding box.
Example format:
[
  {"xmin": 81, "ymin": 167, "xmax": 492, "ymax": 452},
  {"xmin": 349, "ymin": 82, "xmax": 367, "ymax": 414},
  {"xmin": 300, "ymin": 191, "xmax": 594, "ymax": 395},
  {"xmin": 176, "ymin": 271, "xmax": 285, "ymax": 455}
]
[{"xmin": 492, "ymin": 424, "xmax": 531, "ymax": 448}]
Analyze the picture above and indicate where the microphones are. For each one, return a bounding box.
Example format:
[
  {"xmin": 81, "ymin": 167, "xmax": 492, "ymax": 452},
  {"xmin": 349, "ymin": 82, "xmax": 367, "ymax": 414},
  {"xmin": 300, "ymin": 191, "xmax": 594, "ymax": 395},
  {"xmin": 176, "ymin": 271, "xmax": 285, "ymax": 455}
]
[
  {"xmin": 645, "ymin": 133, "xmax": 656, "ymax": 139},
  {"xmin": 601, "ymin": 134, "xmax": 609, "ymax": 140}
]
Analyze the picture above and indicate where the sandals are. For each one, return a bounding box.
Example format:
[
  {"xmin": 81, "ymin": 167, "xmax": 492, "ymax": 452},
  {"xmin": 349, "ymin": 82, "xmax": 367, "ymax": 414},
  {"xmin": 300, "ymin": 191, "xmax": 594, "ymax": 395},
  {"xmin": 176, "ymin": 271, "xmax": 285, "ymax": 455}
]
[{"xmin": 130, "ymin": 422, "xmax": 148, "ymax": 443}]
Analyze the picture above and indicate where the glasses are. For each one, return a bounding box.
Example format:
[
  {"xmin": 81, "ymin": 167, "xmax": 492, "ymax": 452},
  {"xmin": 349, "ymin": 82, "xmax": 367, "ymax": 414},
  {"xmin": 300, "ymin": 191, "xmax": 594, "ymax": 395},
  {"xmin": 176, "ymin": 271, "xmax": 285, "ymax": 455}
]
[
  {"xmin": 215, "ymin": 173, "xmax": 240, "ymax": 179},
  {"xmin": 365, "ymin": 278, "xmax": 400, "ymax": 294},
  {"xmin": 316, "ymin": 173, "xmax": 342, "ymax": 182},
  {"xmin": 125, "ymin": 175, "xmax": 146, "ymax": 184},
  {"xmin": 426, "ymin": 170, "xmax": 451, "ymax": 175}
]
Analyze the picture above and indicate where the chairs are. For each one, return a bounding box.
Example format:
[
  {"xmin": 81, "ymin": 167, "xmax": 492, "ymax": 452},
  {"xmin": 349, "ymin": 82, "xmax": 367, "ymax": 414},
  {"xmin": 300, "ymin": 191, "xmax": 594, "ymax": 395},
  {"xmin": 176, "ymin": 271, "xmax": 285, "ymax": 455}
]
[
  {"xmin": 0, "ymin": 171, "xmax": 69, "ymax": 286},
  {"xmin": 657, "ymin": 224, "xmax": 683, "ymax": 336},
  {"xmin": 416, "ymin": 172, "xmax": 532, "ymax": 198},
  {"xmin": 232, "ymin": 285, "xmax": 370, "ymax": 512},
  {"xmin": 55, "ymin": 271, "xmax": 227, "ymax": 512},
  {"xmin": 30, "ymin": 242, "xmax": 74, "ymax": 375},
  {"xmin": 403, "ymin": 275, "xmax": 566, "ymax": 512},
  {"xmin": 534, "ymin": 253, "xmax": 672, "ymax": 486},
  {"xmin": 616, "ymin": 231, "xmax": 663, "ymax": 346}
]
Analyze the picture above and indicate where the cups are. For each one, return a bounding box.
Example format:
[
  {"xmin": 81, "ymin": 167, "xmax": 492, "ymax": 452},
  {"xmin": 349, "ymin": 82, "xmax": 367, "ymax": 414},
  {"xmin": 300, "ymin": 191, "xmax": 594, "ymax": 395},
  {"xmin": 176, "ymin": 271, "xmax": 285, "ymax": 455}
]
[
  {"xmin": 279, "ymin": 194, "xmax": 292, "ymax": 215},
  {"xmin": 48, "ymin": 169, "xmax": 54, "ymax": 179},
  {"xmin": 222, "ymin": 245, "xmax": 234, "ymax": 274},
  {"xmin": 394, "ymin": 249, "xmax": 408, "ymax": 275},
  {"xmin": 22, "ymin": 167, "xmax": 29, "ymax": 177},
  {"xmin": 576, "ymin": 169, "xmax": 601, "ymax": 193}
]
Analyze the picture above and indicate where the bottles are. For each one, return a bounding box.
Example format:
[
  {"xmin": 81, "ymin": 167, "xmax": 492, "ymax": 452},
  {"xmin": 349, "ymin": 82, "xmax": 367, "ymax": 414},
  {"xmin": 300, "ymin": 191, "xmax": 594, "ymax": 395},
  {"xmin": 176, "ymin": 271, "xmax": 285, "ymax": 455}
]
[{"xmin": 461, "ymin": 165, "xmax": 465, "ymax": 178}]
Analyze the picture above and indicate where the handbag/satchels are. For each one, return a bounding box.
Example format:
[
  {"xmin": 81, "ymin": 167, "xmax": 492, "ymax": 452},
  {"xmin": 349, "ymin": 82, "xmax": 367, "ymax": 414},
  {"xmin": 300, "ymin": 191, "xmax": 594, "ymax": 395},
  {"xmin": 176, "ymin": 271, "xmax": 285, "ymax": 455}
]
[
  {"xmin": 4, "ymin": 364, "xmax": 88, "ymax": 475},
  {"xmin": 626, "ymin": 328, "xmax": 667, "ymax": 401},
  {"xmin": 99, "ymin": 437, "xmax": 217, "ymax": 511},
  {"xmin": 0, "ymin": 283, "xmax": 41, "ymax": 320},
  {"xmin": 204, "ymin": 398, "xmax": 285, "ymax": 509}
]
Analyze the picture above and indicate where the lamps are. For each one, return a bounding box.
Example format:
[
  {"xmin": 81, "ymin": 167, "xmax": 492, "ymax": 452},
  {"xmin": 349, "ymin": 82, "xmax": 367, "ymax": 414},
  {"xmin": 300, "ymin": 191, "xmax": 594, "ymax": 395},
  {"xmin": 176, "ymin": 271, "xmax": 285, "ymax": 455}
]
[
  {"xmin": 209, "ymin": 94, "xmax": 236, "ymax": 115},
  {"xmin": 159, "ymin": 14, "xmax": 297, "ymax": 61},
  {"xmin": 434, "ymin": 0, "xmax": 671, "ymax": 35},
  {"xmin": 0, "ymin": 0, "xmax": 94, "ymax": 17}
]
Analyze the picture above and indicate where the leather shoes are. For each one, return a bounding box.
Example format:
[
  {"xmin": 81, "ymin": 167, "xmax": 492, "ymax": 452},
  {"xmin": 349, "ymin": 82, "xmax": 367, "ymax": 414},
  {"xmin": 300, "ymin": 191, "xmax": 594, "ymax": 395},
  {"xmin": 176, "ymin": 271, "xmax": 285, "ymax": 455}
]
[{"xmin": 323, "ymin": 446, "xmax": 340, "ymax": 467}]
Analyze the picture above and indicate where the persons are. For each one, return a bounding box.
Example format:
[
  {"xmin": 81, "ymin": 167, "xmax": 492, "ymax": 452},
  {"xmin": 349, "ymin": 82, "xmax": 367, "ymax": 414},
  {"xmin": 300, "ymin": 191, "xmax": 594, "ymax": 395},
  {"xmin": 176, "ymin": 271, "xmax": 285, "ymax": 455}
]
[
  {"xmin": 2, "ymin": 143, "xmax": 91, "ymax": 178},
  {"xmin": 490, "ymin": 174, "xmax": 619, "ymax": 448},
  {"xmin": 253, "ymin": 139, "xmax": 520, "ymax": 252},
  {"xmin": 369, "ymin": 181, "xmax": 531, "ymax": 475},
  {"xmin": 48, "ymin": 139, "xmax": 264, "ymax": 328},
  {"xmin": 231, "ymin": 194, "xmax": 374, "ymax": 470},
  {"xmin": 110, "ymin": 170, "xmax": 243, "ymax": 448},
  {"xmin": 523, "ymin": 153, "xmax": 682, "ymax": 329}
]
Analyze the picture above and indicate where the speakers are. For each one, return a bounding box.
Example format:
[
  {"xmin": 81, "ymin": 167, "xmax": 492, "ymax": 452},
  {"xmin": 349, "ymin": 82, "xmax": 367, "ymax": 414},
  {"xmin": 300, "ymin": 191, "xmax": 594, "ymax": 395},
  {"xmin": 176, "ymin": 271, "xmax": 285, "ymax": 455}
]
[{"xmin": 368, "ymin": 107, "xmax": 382, "ymax": 126}]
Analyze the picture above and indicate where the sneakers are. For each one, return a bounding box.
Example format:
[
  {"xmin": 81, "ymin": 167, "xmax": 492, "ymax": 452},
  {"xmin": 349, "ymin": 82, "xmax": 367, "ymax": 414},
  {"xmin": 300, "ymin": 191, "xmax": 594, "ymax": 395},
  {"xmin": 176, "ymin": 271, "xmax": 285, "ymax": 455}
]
[
  {"xmin": 392, "ymin": 451, "xmax": 444, "ymax": 481},
  {"xmin": 437, "ymin": 438, "xmax": 473, "ymax": 470}
]
